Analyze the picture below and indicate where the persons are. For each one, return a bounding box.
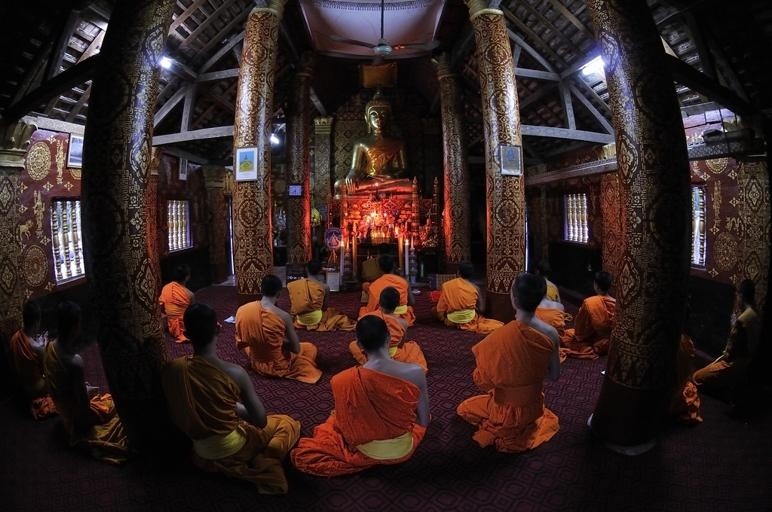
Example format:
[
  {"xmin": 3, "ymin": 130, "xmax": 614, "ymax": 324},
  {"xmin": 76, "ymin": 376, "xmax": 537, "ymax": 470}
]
[
  {"xmin": 692, "ymin": 278, "xmax": 764, "ymax": 407},
  {"xmin": 234, "ymin": 258, "xmax": 347, "ymax": 383},
  {"xmin": 433, "ymin": 266, "xmax": 482, "ymax": 329},
  {"xmin": 455, "ymin": 270, "xmax": 560, "ymax": 440},
  {"xmin": 535, "ymin": 266, "xmax": 617, "ymax": 358},
  {"xmin": 329, "ymin": 314, "xmax": 432, "ymax": 465},
  {"xmin": 44, "ymin": 298, "xmax": 116, "ymax": 437},
  {"xmin": 9, "ymin": 298, "xmax": 47, "ymax": 397},
  {"xmin": 158, "ymin": 261, "xmax": 199, "ymax": 343},
  {"xmin": 350, "ymin": 256, "xmax": 420, "ymax": 364},
  {"xmin": 160, "ymin": 302, "xmax": 302, "ymax": 494},
  {"xmin": 331, "ymin": 90, "xmax": 419, "ymax": 198}
]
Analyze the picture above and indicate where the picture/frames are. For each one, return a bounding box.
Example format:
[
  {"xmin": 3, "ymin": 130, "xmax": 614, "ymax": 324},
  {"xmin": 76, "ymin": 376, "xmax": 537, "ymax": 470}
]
[
  {"xmin": 499, "ymin": 144, "xmax": 524, "ymax": 176},
  {"xmin": 234, "ymin": 145, "xmax": 259, "ymax": 182}
]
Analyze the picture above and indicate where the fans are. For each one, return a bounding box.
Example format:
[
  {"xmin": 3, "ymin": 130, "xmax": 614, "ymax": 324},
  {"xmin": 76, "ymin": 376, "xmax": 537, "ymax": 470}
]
[{"xmin": 328, "ymin": 0, "xmax": 439, "ymax": 65}]
